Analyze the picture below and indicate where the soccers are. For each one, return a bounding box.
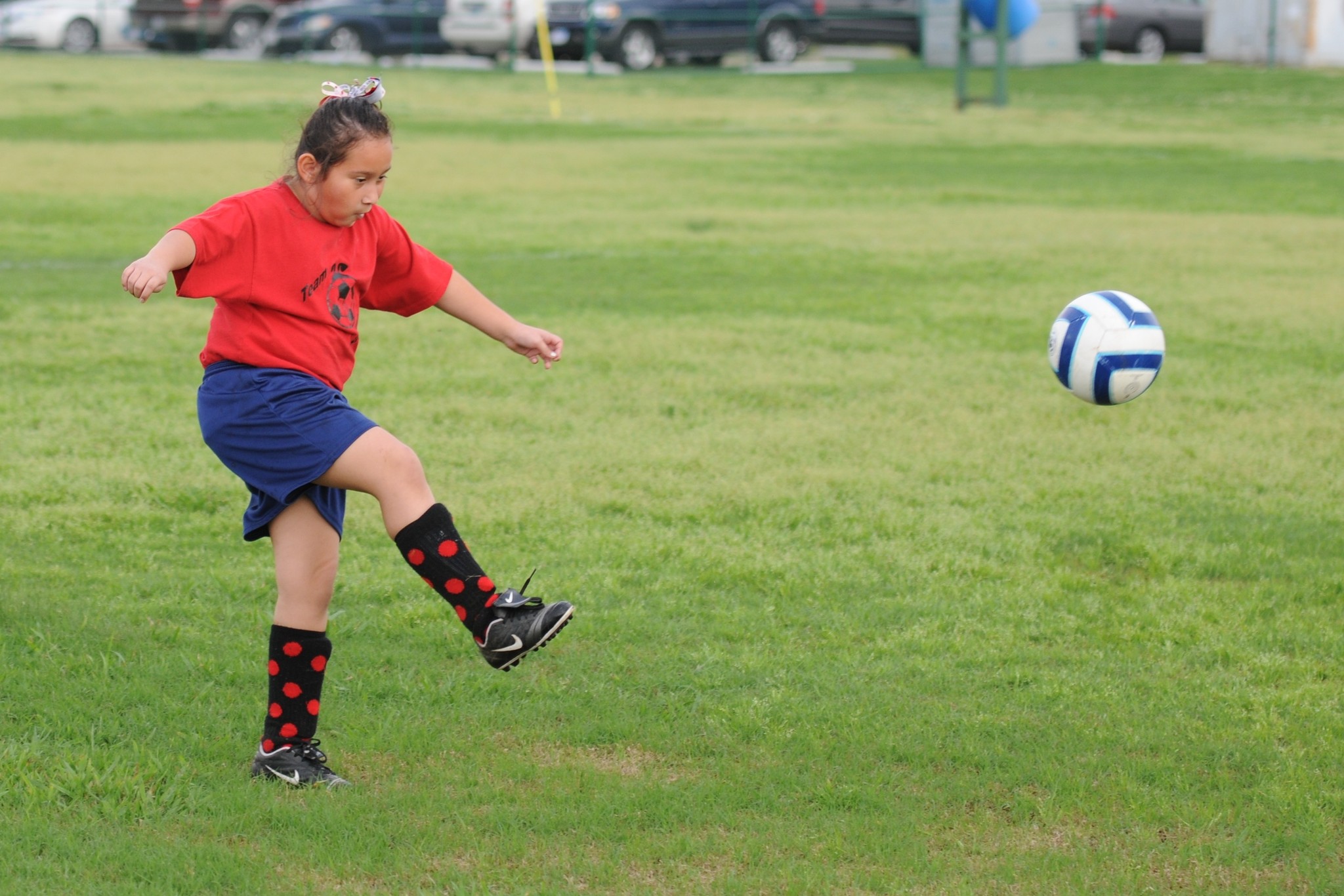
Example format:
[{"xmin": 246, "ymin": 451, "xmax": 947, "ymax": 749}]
[{"xmin": 1047, "ymin": 289, "xmax": 1165, "ymax": 406}]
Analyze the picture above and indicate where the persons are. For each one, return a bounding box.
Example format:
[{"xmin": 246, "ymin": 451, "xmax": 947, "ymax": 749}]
[{"xmin": 118, "ymin": 76, "xmax": 577, "ymax": 793}]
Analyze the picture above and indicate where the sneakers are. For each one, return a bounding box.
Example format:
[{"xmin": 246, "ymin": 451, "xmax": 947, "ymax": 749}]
[
  {"xmin": 251, "ymin": 736, "xmax": 354, "ymax": 799},
  {"xmin": 472, "ymin": 565, "xmax": 576, "ymax": 675}
]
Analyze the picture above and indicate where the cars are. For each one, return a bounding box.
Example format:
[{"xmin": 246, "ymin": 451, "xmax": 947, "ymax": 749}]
[{"xmin": 1, "ymin": 1, "xmax": 1210, "ymax": 67}]
